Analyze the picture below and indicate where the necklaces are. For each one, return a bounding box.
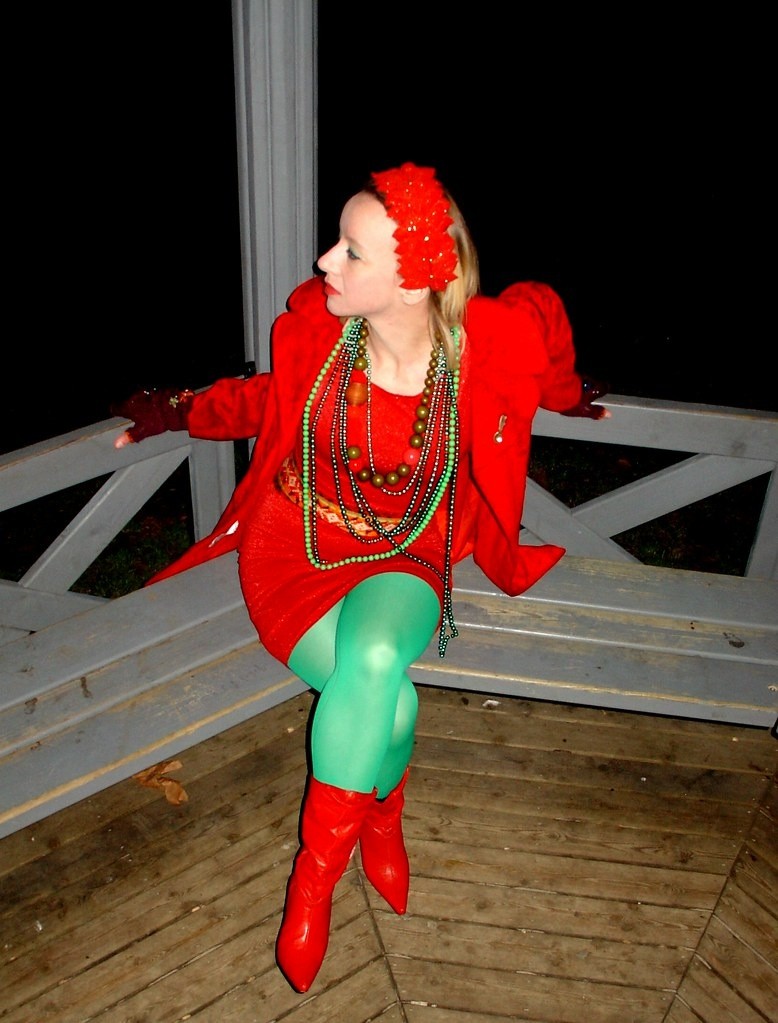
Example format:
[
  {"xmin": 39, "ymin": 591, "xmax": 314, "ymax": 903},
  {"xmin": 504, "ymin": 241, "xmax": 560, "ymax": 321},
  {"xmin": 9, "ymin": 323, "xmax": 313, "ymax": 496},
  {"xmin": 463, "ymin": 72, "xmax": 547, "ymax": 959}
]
[{"xmin": 302, "ymin": 321, "xmax": 460, "ymax": 659}]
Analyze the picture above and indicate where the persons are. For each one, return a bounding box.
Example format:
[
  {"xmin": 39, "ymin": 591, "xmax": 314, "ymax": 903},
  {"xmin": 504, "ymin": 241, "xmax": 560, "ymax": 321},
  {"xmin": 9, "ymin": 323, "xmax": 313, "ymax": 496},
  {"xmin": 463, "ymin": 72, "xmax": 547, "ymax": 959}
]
[{"xmin": 110, "ymin": 162, "xmax": 612, "ymax": 993}]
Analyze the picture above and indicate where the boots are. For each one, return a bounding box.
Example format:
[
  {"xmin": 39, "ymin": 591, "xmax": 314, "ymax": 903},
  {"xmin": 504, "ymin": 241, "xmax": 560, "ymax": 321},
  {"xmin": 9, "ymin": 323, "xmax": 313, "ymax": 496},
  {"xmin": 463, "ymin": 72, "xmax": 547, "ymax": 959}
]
[
  {"xmin": 357, "ymin": 767, "xmax": 409, "ymax": 917},
  {"xmin": 276, "ymin": 775, "xmax": 377, "ymax": 994}
]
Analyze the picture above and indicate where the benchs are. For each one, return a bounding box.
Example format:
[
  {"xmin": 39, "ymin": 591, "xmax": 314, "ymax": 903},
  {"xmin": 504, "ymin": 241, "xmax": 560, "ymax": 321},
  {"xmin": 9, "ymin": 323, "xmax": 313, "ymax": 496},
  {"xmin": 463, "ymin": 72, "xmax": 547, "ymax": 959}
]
[
  {"xmin": 0, "ymin": 549, "xmax": 311, "ymax": 839},
  {"xmin": 403, "ymin": 553, "xmax": 778, "ymax": 729}
]
